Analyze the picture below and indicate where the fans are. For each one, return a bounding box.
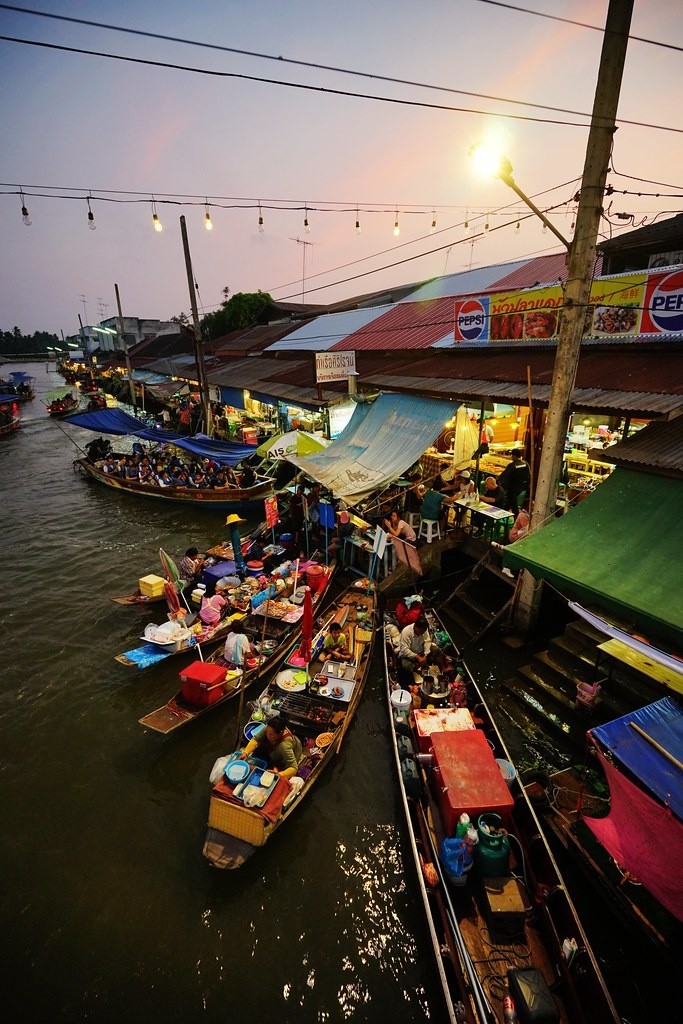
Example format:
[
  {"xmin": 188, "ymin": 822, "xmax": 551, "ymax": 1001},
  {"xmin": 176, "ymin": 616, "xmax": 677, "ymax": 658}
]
[{"xmin": 444, "ymin": 431, "xmax": 456, "ymax": 453}]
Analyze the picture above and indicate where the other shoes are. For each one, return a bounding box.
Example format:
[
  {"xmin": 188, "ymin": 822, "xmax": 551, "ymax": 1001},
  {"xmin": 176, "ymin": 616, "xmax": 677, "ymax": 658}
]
[{"xmin": 472, "ymin": 532, "xmax": 484, "ymax": 538}]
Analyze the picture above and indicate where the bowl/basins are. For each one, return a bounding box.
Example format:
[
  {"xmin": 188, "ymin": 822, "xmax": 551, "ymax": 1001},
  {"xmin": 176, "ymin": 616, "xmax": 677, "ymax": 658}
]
[
  {"xmin": 226, "ymin": 759, "xmax": 250, "ymax": 784},
  {"xmin": 257, "ymin": 640, "xmax": 278, "ymax": 651}
]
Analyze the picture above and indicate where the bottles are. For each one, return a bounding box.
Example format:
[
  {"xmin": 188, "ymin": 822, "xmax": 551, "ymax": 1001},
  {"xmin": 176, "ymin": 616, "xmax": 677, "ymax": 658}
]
[{"xmin": 465, "ymin": 491, "xmax": 476, "ymax": 503}]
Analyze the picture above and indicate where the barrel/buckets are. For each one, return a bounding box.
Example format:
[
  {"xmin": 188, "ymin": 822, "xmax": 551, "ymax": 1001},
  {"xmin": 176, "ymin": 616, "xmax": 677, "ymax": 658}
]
[
  {"xmin": 306, "ymin": 565, "xmax": 325, "ymax": 591},
  {"xmin": 247, "ymin": 560, "xmax": 266, "ymax": 576},
  {"xmin": 391, "ymin": 689, "xmax": 412, "ymax": 716},
  {"xmin": 495, "ymin": 758, "xmax": 515, "ymax": 790}
]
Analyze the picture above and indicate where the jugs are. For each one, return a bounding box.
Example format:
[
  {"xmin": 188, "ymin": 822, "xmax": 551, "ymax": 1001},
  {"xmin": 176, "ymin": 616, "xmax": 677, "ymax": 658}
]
[{"xmin": 423, "ymin": 674, "xmax": 449, "ymax": 694}]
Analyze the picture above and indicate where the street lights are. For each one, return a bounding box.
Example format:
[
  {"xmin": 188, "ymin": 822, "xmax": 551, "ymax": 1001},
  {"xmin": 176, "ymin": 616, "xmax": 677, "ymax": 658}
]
[{"xmin": 469, "ymin": 103, "xmax": 617, "ymax": 639}]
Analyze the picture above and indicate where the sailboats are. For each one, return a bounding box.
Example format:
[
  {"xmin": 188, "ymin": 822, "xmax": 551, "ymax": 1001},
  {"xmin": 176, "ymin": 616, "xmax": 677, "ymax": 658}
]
[
  {"xmin": 40, "ymin": 283, "xmax": 159, "ymax": 428},
  {"xmin": 52, "ymin": 214, "xmax": 277, "ymax": 511}
]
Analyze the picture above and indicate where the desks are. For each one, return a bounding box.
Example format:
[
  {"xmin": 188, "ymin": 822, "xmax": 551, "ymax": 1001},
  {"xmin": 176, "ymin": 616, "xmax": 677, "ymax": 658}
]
[
  {"xmin": 452, "ymin": 498, "xmax": 515, "ymax": 546},
  {"xmin": 341, "ymin": 537, "xmax": 380, "ymax": 579},
  {"xmin": 206, "ymin": 546, "xmax": 235, "ymax": 560},
  {"xmin": 566, "ymin": 459, "xmax": 611, "ymax": 471},
  {"xmin": 249, "ymin": 601, "xmax": 305, "ymax": 624},
  {"xmin": 567, "ymin": 469, "xmax": 600, "ymax": 479},
  {"xmin": 419, "ymin": 453, "xmax": 454, "ymax": 487},
  {"xmin": 431, "ymin": 730, "xmax": 514, "ymax": 833},
  {"xmin": 469, "ymin": 466, "xmax": 503, "ymax": 482}
]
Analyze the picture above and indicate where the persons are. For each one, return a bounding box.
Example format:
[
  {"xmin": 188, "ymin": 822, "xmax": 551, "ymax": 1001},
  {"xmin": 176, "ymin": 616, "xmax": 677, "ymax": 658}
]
[
  {"xmin": 238, "ymin": 716, "xmax": 303, "ymax": 780},
  {"xmin": 226, "ymin": 619, "xmax": 255, "ymax": 666},
  {"xmin": 251, "ymin": 536, "xmax": 268, "ymax": 561},
  {"xmin": 156, "ymin": 396, "xmax": 225, "ymax": 434},
  {"xmin": 0, "ymin": 380, "xmax": 30, "ymax": 396},
  {"xmin": 318, "ymin": 623, "xmax": 351, "ymax": 662},
  {"xmin": 199, "ymin": 581, "xmax": 230, "ymax": 625},
  {"xmin": 179, "ymin": 547, "xmax": 205, "ymax": 581},
  {"xmin": 46, "ymin": 394, "xmax": 74, "ymax": 411},
  {"xmin": 400, "ymin": 617, "xmax": 443, "ymax": 685},
  {"xmin": 289, "ymin": 449, "xmax": 534, "ymax": 562},
  {"xmin": 102, "ymin": 446, "xmax": 257, "ymax": 491},
  {"xmin": 0, "ymin": 406, "xmax": 16, "ymax": 426}
]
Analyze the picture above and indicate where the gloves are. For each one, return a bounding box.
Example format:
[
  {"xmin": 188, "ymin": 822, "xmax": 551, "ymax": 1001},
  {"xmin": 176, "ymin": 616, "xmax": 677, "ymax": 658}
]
[
  {"xmin": 268, "ymin": 767, "xmax": 297, "ymax": 779},
  {"xmin": 238, "ymin": 739, "xmax": 262, "ymax": 760}
]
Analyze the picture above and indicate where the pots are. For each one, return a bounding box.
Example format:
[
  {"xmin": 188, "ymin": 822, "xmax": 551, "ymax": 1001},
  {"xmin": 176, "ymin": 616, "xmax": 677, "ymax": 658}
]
[{"xmin": 216, "ymin": 574, "xmax": 241, "ymax": 589}]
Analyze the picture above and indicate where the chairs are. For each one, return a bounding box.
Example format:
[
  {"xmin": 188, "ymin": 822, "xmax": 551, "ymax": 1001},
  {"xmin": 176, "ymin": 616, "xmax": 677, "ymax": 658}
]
[{"xmin": 558, "ymin": 485, "xmax": 566, "ymax": 495}]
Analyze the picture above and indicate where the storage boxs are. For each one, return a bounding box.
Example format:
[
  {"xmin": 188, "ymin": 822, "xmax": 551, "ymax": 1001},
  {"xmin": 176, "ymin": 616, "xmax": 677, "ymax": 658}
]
[
  {"xmin": 413, "ymin": 708, "xmax": 476, "ymax": 752},
  {"xmin": 284, "ymin": 644, "xmax": 316, "ymax": 670},
  {"xmin": 139, "ymin": 574, "xmax": 165, "ymax": 598},
  {"xmin": 178, "ymin": 661, "xmax": 227, "ymax": 705}
]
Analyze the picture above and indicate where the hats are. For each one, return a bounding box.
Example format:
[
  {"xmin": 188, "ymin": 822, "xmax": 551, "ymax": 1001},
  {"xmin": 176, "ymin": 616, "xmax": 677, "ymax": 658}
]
[
  {"xmin": 457, "ymin": 470, "xmax": 470, "ymax": 479},
  {"xmin": 508, "ymin": 449, "xmax": 522, "ymax": 458},
  {"xmin": 222, "ymin": 514, "xmax": 246, "ymax": 528},
  {"xmin": 341, "ymin": 512, "xmax": 350, "ymax": 524}
]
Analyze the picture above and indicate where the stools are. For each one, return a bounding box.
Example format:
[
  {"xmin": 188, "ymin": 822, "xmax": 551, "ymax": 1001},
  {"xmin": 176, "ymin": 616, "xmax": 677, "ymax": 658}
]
[
  {"xmin": 406, "ymin": 512, "xmax": 421, "ymax": 528},
  {"xmin": 418, "ymin": 520, "xmax": 441, "ymax": 543}
]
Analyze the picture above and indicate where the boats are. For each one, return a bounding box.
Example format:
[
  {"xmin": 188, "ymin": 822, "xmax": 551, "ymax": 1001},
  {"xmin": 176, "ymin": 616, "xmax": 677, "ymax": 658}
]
[
  {"xmin": 138, "ymin": 561, "xmax": 338, "ymax": 737},
  {"xmin": 382, "ymin": 593, "xmax": 626, "ymax": 1024},
  {"xmin": 112, "ymin": 553, "xmax": 320, "ymax": 669},
  {"xmin": 109, "ymin": 524, "xmax": 278, "ymax": 607},
  {"xmin": 203, "ymin": 576, "xmax": 377, "ymax": 874},
  {"xmin": 0, "ymin": 370, "xmax": 37, "ymax": 436}
]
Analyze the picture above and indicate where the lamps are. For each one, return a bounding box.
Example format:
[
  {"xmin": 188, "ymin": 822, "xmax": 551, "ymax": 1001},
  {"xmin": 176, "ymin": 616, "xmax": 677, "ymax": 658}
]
[
  {"xmin": 489, "ymin": 418, "xmax": 500, "ymax": 425},
  {"xmin": 509, "ymin": 421, "xmax": 520, "ymax": 429}
]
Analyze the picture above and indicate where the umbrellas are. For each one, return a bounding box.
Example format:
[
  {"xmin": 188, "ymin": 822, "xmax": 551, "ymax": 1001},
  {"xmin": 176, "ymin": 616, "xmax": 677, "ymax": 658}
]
[
  {"xmin": 257, "ymin": 429, "xmax": 332, "ymax": 492},
  {"xmin": 299, "ymin": 585, "xmax": 312, "ymax": 675},
  {"xmin": 157, "ymin": 548, "xmax": 192, "ymax": 632}
]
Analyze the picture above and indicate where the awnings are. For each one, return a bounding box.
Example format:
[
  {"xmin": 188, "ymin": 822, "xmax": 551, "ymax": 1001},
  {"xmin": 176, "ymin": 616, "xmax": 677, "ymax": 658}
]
[
  {"xmin": 284, "ymin": 394, "xmax": 459, "ymax": 504},
  {"xmin": 490, "ymin": 467, "xmax": 683, "ymax": 631}
]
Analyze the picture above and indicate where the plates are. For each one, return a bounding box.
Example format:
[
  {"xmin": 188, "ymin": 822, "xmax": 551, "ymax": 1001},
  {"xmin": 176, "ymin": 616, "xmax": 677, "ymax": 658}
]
[
  {"xmin": 330, "ymin": 687, "xmax": 344, "ymax": 698},
  {"xmin": 319, "ymin": 687, "xmax": 330, "ymax": 697}
]
